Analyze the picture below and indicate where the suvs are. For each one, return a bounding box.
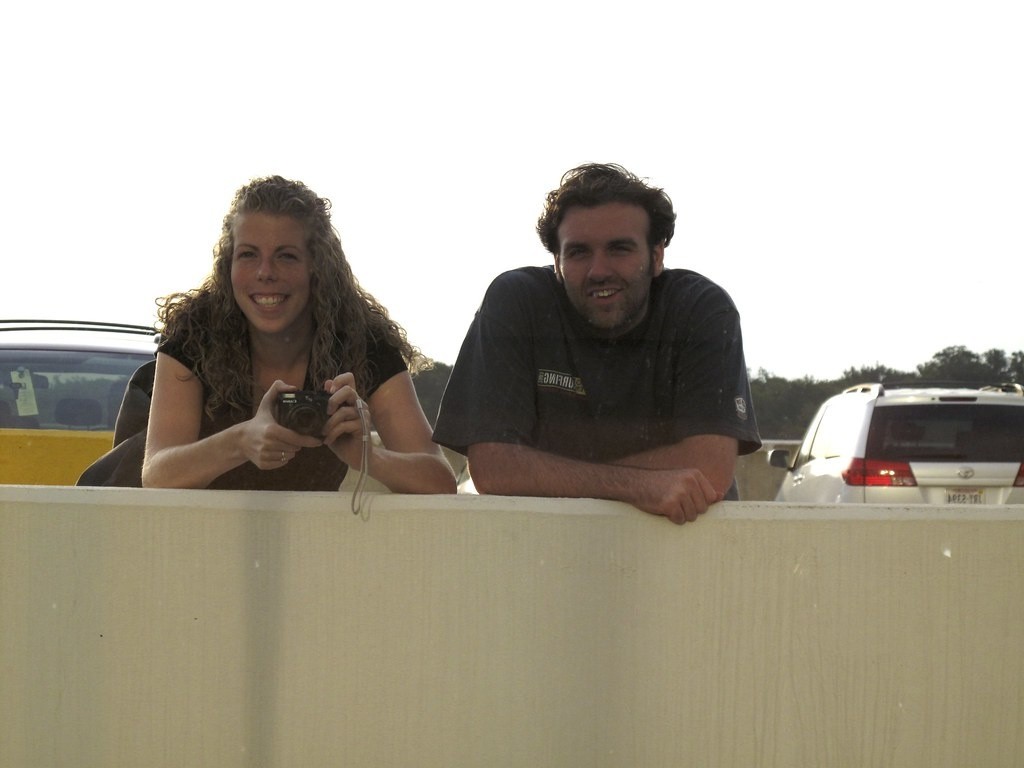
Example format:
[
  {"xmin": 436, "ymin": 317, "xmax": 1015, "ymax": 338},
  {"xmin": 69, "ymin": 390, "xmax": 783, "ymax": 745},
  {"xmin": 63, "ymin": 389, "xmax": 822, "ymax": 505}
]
[{"xmin": 766, "ymin": 382, "xmax": 1024, "ymax": 507}]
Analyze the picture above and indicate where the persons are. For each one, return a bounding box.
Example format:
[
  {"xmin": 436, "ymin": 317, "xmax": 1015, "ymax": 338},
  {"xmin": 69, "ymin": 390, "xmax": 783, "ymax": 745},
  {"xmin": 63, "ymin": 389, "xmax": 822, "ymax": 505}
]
[
  {"xmin": 74, "ymin": 176, "xmax": 458, "ymax": 496},
  {"xmin": 432, "ymin": 163, "xmax": 762, "ymax": 525}
]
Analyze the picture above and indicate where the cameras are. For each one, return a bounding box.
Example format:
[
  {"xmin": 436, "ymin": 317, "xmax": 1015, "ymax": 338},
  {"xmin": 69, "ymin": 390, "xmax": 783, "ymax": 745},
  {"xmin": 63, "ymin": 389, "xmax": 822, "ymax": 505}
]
[{"xmin": 274, "ymin": 391, "xmax": 348, "ymax": 440}]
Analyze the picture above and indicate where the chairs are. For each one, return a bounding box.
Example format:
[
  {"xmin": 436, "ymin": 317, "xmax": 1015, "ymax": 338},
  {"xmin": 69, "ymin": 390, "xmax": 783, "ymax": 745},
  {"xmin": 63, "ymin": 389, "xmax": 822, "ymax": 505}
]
[
  {"xmin": 54, "ymin": 397, "xmax": 104, "ymax": 431},
  {"xmin": 891, "ymin": 424, "xmax": 925, "ymax": 455}
]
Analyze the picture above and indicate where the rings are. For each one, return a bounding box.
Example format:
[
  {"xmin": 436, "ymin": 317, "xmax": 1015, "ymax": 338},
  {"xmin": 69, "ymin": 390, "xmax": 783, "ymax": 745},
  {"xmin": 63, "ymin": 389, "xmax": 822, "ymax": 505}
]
[{"xmin": 281, "ymin": 451, "xmax": 287, "ymax": 462}]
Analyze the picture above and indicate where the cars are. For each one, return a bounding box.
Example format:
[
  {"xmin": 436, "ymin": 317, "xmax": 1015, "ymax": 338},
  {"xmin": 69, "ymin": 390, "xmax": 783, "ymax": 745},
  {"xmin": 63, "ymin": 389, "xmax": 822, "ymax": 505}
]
[{"xmin": 0, "ymin": 317, "xmax": 390, "ymax": 495}]
